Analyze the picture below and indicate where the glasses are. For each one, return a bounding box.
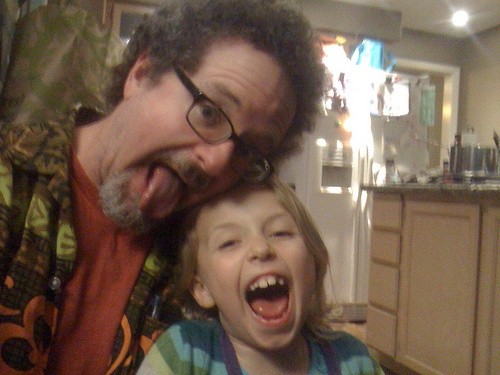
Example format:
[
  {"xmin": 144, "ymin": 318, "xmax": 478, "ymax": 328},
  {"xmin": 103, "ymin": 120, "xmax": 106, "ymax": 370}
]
[{"xmin": 174, "ymin": 63, "xmax": 271, "ymax": 185}]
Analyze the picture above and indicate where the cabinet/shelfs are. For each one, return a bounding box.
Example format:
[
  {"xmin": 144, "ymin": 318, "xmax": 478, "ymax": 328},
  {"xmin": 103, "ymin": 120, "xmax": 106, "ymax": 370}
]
[{"xmin": 395, "ymin": 200, "xmax": 479, "ymax": 375}]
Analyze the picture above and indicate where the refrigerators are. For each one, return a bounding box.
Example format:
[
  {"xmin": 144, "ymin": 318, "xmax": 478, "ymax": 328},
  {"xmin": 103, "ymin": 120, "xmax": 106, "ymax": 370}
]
[{"xmin": 278, "ymin": 65, "xmax": 432, "ymax": 322}]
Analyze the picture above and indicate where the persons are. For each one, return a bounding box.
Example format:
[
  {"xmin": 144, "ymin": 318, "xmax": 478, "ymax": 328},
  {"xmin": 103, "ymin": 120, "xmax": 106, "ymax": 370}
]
[
  {"xmin": 0, "ymin": 0, "xmax": 324, "ymax": 375},
  {"xmin": 133, "ymin": 164, "xmax": 385, "ymax": 375}
]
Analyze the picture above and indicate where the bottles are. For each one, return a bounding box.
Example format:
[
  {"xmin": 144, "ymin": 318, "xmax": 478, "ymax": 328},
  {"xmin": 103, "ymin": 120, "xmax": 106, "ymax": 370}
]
[{"xmin": 451, "ymin": 135, "xmax": 463, "ymax": 184}]
